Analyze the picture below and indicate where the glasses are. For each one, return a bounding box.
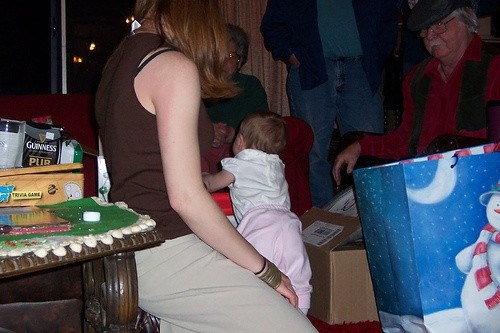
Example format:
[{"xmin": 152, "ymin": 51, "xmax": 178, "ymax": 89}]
[
  {"xmin": 227, "ymin": 51, "xmax": 241, "ymax": 64},
  {"xmin": 419, "ymin": 16, "xmax": 456, "ymax": 38}
]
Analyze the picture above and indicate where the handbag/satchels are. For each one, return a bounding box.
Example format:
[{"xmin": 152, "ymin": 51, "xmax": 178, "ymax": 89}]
[{"xmin": 352, "ymin": 142, "xmax": 500, "ymax": 333}]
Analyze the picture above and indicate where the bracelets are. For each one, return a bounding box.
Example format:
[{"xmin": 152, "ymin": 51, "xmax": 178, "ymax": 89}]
[{"xmin": 254, "ymin": 256, "xmax": 281, "ymax": 290}]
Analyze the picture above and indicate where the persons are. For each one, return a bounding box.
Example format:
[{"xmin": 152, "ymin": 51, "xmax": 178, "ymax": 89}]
[
  {"xmin": 93, "ymin": 0, "xmax": 316, "ymax": 333},
  {"xmin": 200, "ymin": 22, "xmax": 270, "ymax": 178},
  {"xmin": 201, "ymin": 112, "xmax": 312, "ymax": 317},
  {"xmin": 260, "ymin": 0, "xmax": 406, "ymax": 208},
  {"xmin": 332, "ymin": 0, "xmax": 500, "ymax": 190}
]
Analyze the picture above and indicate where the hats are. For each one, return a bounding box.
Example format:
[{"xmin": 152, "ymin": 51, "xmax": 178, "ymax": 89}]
[{"xmin": 407, "ymin": 0, "xmax": 467, "ymax": 31}]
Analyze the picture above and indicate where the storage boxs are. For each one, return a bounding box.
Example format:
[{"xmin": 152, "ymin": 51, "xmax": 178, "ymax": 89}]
[{"xmin": 301, "ymin": 206, "xmax": 381, "ymax": 326}]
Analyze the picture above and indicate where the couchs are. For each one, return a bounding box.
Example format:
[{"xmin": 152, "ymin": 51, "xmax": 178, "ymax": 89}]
[{"xmin": 1, "ymin": 93, "xmax": 314, "ymax": 218}]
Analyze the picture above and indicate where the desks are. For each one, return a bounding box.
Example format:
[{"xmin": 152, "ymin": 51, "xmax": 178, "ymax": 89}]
[{"xmin": 0, "ymin": 210, "xmax": 164, "ymax": 333}]
